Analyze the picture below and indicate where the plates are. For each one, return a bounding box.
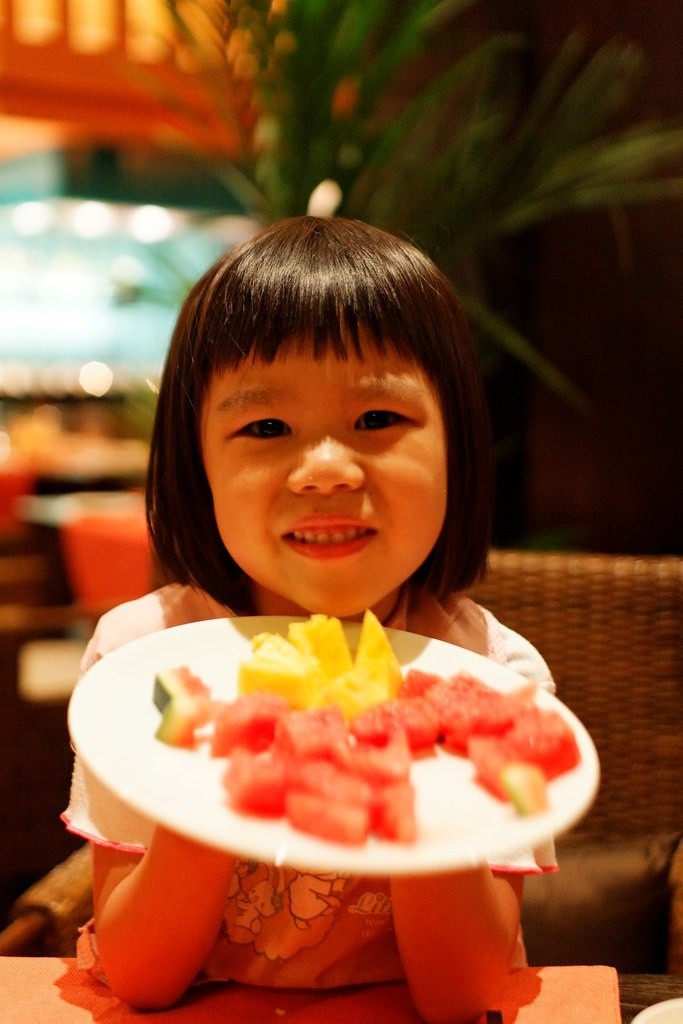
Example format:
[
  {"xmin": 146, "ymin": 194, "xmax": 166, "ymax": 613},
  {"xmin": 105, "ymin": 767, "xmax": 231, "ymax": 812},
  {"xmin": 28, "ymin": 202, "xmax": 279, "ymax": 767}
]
[{"xmin": 66, "ymin": 614, "xmax": 601, "ymax": 875}]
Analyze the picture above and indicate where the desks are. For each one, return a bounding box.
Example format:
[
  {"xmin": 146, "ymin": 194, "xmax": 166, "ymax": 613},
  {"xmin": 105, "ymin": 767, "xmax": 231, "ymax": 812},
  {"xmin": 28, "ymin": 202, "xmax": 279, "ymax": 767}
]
[{"xmin": 0, "ymin": 956, "xmax": 624, "ymax": 1024}]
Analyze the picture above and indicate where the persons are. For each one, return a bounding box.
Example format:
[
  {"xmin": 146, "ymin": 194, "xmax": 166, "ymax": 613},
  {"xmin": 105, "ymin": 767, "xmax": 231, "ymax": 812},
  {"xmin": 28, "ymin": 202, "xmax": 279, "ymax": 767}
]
[{"xmin": 59, "ymin": 220, "xmax": 563, "ymax": 1024}]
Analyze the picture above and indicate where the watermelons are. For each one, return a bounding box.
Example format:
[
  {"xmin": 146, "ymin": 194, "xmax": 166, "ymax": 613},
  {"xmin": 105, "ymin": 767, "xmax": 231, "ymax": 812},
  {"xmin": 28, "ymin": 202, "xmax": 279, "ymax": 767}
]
[{"xmin": 152, "ymin": 666, "xmax": 580, "ymax": 846}]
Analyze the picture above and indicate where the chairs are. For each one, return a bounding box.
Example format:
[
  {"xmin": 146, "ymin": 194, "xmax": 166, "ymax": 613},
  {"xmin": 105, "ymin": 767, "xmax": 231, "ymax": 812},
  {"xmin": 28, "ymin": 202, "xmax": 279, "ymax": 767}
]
[{"xmin": 0, "ymin": 552, "xmax": 683, "ymax": 979}]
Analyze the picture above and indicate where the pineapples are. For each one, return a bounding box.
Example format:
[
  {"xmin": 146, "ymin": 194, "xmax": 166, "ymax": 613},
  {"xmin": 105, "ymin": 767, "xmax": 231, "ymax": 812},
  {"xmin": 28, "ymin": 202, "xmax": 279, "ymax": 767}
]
[{"xmin": 234, "ymin": 607, "xmax": 402, "ymax": 726}]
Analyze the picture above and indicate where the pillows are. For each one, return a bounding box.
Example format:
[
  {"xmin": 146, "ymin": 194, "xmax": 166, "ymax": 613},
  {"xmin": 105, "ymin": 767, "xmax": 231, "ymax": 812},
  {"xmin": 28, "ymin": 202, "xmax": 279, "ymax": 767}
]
[{"xmin": 519, "ymin": 829, "xmax": 683, "ymax": 976}]
